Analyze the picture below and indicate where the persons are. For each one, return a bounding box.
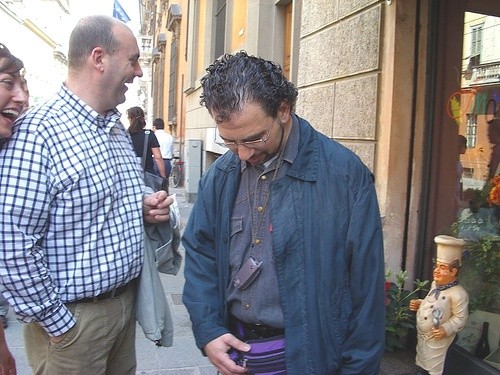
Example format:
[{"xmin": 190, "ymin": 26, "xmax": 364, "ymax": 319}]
[
  {"xmin": 182, "ymin": 50, "xmax": 388, "ymax": 375},
  {"xmin": 0, "ymin": 16, "xmax": 175, "ymax": 375},
  {"xmin": 487, "ymin": 119, "xmax": 500, "ymax": 180},
  {"xmin": 409, "ymin": 235, "xmax": 469, "ymax": 375},
  {"xmin": 457, "ymin": 135, "xmax": 470, "ymax": 209},
  {"xmin": 152, "ymin": 119, "xmax": 173, "ymax": 182},
  {"xmin": 0, "ymin": 44, "xmax": 29, "ymax": 375},
  {"xmin": 124, "ymin": 107, "xmax": 166, "ymax": 181}
]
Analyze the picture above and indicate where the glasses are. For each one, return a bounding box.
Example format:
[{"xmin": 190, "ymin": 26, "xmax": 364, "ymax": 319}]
[{"xmin": 214, "ymin": 115, "xmax": 274, "ymax": 148}]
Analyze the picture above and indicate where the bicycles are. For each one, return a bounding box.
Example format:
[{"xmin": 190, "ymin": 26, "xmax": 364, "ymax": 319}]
[{"xmin": 170, "ymin": 156, "xmax": 184, "ymax": 185}]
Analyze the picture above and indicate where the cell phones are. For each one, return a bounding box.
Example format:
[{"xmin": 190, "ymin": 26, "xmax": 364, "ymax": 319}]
[{"xmin": 235, "ymin": 258, "xmax": 263, "ymax": 288}]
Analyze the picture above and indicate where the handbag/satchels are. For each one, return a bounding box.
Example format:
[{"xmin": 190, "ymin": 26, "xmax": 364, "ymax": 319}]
[{"xmin": 145, "ymin": 172, "xmax": 168, "ymax": 192}]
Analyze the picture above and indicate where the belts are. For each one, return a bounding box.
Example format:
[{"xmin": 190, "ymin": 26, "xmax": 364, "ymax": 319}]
[{"xmin": 71, "ymin": 284, "xmax": 126, "ymax": 303}]
